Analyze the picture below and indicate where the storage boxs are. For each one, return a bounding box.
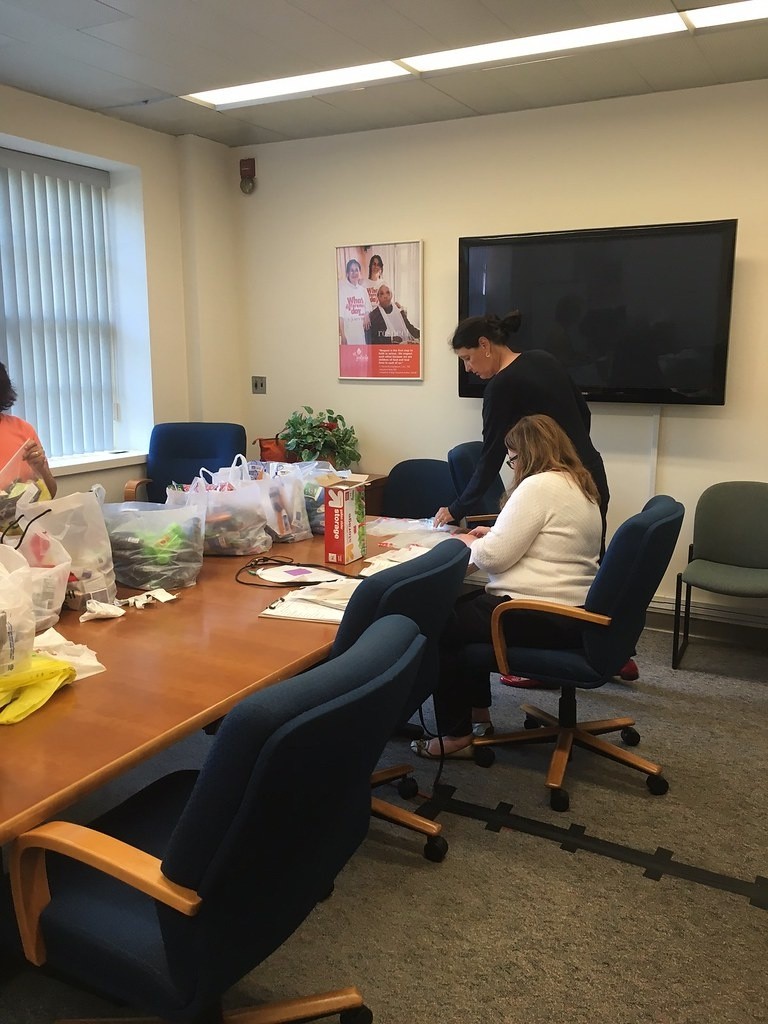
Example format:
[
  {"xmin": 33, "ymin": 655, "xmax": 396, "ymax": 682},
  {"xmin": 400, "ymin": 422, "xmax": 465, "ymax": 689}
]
[{"xmin": 316, "ymin": 471, "xmax": 379, "ymax": 565}]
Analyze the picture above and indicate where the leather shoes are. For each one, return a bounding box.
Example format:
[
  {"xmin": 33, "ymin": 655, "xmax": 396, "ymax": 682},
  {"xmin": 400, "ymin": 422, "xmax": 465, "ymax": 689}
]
[
  {"xmin": 614, "ymin": 658, "xmax": 639, "ymax": 681},
  {"xmin": 500, "ymin": 676, "xmax": 544, "ymax": 688}
]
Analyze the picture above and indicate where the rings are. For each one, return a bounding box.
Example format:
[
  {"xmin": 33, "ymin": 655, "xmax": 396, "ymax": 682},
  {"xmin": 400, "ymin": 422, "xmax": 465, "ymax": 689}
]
[
  {"xmin": 36, "ymin": 452, "xmax": 40, "ymax": 456},
  {"xmin": 437, "ymin": 519, "xmax": 439, "ymax": 522}
]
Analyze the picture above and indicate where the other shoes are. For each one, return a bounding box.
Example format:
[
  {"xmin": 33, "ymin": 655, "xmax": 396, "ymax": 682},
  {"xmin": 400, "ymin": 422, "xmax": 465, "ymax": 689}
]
[
  {"xmin": 471, "ymin": 721, "xmax": 494, "ymax": 738},
  {"xmin": 410, "ymin": 737, "xmax": 474, "ymax": 759}
]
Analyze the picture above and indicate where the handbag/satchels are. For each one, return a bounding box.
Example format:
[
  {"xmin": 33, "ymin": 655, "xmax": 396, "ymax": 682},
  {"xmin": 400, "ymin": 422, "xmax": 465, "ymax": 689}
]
[
  {"xmin": 305, "ymin": 459, "xmax": 334, "ymax": 534},
  {"xmin": 0, "ymin": 545, "xmax": 34, "ymax": 677},
  {"xmin": 166, "ymin": 468, "xmax": 272, "ymax": 558},
  {"xmin": 230, "ymin": 460, "xmax": 314, "ymax": 543},
  {"xmin": 89, "ymin": 478, "xmax": 210, "ymax": 592},
  {"xmin": 16, "ymin": 483, "xmax": 119, "ymax": 610},
  {"xmin": 197, "ymin": 455, "xmax": 295, "ymax": 545},
  {"xmin": 1, "ymin": 529, "xmax": 71, "ymax": 630}
]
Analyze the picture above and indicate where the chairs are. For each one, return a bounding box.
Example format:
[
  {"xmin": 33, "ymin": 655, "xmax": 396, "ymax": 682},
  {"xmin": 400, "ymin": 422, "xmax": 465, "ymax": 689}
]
[
  {"xmin": 11, "ymin": 615, "xmax": 428, "ymax": 1024},
  {"xmin": 471, "ymin": 495, "xmax": 685, "ymax": 812},
  {"xmin": 124, "ymin": 422, "xmax": 247, "ymax": 504},
  {"xmin": 382, "ymin": 441, "xmax": 506, "ymax": 528},
  {"xmin": 672, "ymin": 481, "xmax": 768, "ymax": 668},
  {"xmin": 328, "ymin": 538, "xmax": 471, "ymax": 861}
]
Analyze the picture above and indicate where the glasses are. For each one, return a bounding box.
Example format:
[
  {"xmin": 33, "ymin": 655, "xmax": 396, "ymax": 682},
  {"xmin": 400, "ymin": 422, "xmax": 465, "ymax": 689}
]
[{"xmin": 506, "ymin": 453, "xmax": 519, "ymax": 469}]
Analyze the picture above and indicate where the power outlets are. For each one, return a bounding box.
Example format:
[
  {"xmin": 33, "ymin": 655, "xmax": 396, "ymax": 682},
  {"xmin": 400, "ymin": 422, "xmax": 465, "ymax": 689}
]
[{"xmin": 251, "ymin": 376, "xmax": 266, "ymax": 395}]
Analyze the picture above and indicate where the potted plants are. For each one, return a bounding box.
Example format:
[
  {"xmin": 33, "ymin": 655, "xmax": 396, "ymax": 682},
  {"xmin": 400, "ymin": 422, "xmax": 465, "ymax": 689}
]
[{"xmin": 282, "ymin": 406, "xmax": 360, "ymax": 470}]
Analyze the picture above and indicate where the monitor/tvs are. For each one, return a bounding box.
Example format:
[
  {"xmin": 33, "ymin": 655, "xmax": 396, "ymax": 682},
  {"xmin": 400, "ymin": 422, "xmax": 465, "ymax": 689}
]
[{"xmin": 458, "ymin": 218, "xmax": 738, "ymax": 404}]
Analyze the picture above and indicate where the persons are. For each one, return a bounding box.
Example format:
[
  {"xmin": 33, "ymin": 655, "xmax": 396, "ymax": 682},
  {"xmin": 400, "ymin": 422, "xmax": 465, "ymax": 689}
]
[
  {"xmin": 432, "ymin": 310, "xmax": 640, "ymax": 690},
  {"xmin": 0, "ymin": 361, "xmax": 57, "ymax": 499},
  {"xmin": 409, "ymin": 414, "xmax": 602, "ymax": 762},
  {"xmin": 339, "ymin": 255, "xmax": 420, "ymax": 344}
]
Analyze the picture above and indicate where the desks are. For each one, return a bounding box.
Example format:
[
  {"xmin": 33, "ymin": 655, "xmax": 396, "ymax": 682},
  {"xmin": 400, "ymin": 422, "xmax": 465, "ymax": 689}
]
[
  {"xmin": 356, "ymin": 471, "xmax": 388, "ymax": 517},
  {"xmin": 0, "ymin": 515, "xmax": 480, "ymax": 845}
]
